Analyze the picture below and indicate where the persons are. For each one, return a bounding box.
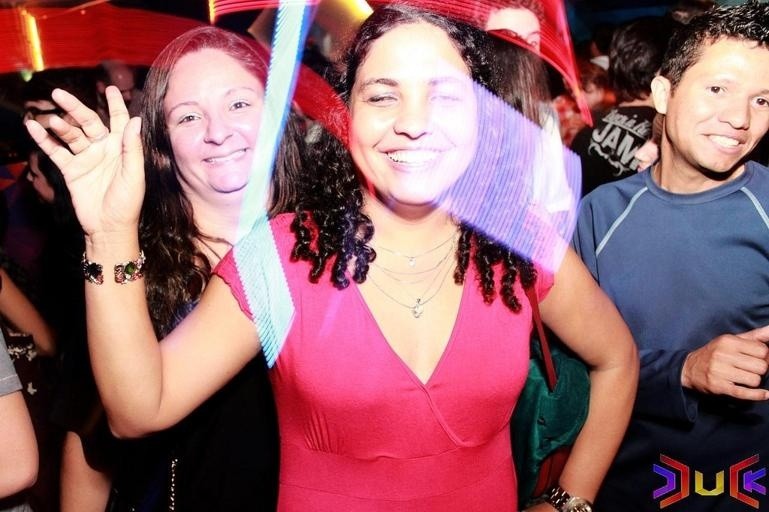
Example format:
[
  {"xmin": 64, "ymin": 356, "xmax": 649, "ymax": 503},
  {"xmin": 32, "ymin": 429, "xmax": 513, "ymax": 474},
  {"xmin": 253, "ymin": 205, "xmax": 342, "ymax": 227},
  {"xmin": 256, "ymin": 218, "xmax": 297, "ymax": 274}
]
[{"xmin": 0, "ymin": 0, "xmax": 769, "ymax": 512}]
